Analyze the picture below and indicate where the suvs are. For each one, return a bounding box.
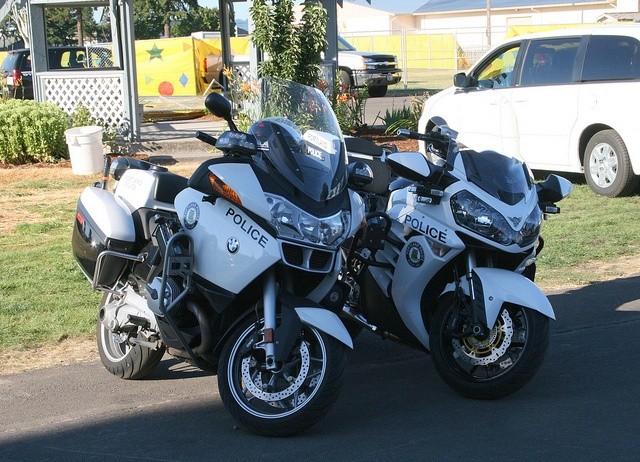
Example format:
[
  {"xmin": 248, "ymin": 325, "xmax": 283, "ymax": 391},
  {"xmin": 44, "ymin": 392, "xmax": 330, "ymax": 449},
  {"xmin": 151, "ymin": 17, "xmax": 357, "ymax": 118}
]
[
  {"xmin": 1, "ymin": 46, "xmax": 111, "ymax": 101},
  {"xmin": 309, "ymin": 33, "xmax": 403, "ymax": 99}
]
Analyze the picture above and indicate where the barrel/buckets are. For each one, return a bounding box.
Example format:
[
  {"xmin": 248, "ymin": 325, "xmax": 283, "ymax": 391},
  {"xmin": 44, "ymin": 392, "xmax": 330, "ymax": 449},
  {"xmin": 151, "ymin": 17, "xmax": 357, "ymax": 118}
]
[{"xmin": 64, "ymin": 124, "xmax": 105, "ymax": 176}]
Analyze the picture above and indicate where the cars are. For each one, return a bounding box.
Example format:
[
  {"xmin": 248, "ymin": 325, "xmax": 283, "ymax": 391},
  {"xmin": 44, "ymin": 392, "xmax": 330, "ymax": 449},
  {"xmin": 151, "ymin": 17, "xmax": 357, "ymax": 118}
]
[{"xmin": 415, "ymin": 24, "xmax": 639, "ymax": 199}]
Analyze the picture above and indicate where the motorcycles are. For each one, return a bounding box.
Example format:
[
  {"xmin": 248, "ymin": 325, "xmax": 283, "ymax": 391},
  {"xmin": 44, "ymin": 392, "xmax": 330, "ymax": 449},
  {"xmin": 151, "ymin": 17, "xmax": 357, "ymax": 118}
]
[
  {"xmin": 70, "ymin": 74, "xmax": 376, "ymax": 439},
  {"xmin": 345, "ymin": 124, "xmax": 574, "ymax": 402}
]
